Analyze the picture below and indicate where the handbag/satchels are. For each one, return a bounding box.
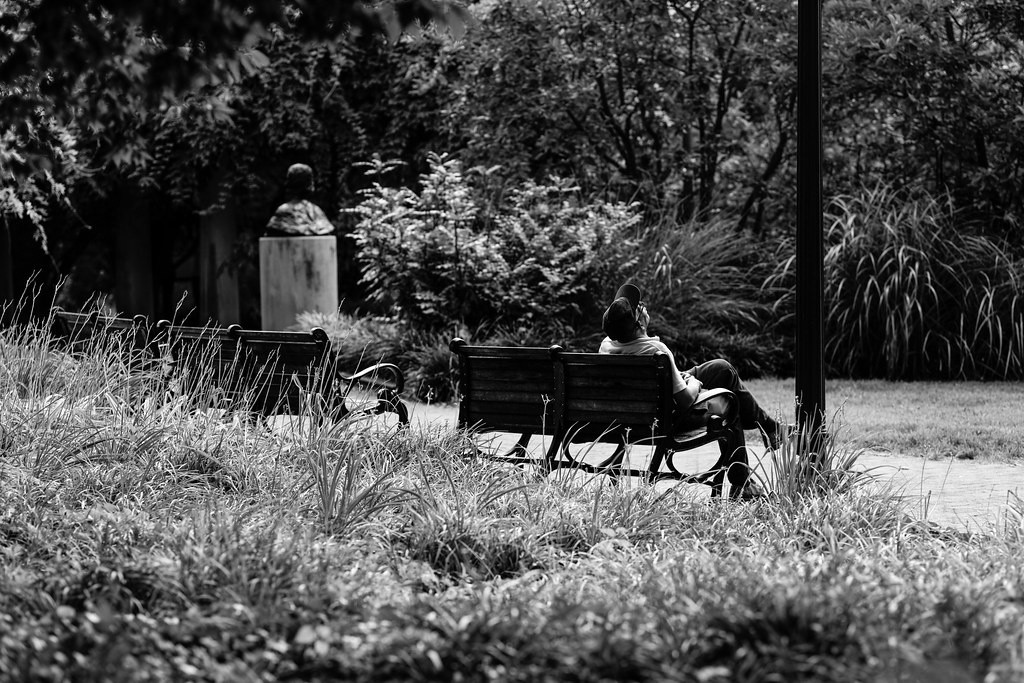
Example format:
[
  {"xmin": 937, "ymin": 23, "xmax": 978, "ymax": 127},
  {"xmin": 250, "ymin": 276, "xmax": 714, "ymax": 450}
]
[{"xmin": 685, "ymin": 387, "xmax": 739, "ymax": 433}]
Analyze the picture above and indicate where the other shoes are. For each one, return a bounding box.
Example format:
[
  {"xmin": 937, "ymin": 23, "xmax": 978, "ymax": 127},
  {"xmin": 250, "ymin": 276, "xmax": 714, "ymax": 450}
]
[
  {"xmin": 762, "ymin": 425, "xmax": 798, "ymax": 451},
  {"xmin": 730, "ymin": 478, "xmax": 765, "ymax": 496}
]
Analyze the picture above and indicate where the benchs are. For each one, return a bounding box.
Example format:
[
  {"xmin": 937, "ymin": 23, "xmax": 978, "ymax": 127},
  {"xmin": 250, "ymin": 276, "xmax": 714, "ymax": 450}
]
[
  {"xmin": 157, "ymin": 320, "xmax": 411, "ymax": 437},
  {"xmin": 449, "ymin": 338, "xmax": 753, "ymax": 503},
  {"xmin": 50, "ymin": 306, "xmax": 172, "ymax": 411}
]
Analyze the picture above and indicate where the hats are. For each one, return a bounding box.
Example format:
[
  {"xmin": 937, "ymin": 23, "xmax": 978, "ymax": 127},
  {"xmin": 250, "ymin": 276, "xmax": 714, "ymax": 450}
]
[{"xmin": 603, "ymin": 284, "xmax": 641, "ymax": 341}]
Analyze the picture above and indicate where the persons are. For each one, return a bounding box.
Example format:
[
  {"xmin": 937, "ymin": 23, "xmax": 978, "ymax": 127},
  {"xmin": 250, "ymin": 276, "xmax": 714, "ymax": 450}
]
[
  {"xmin": 598, "ymin": 284, "xmax": 795, "ymax": 502},
  {"xmin": 266, "ymin": 163, "xmax": 335, "ymax": 236}
]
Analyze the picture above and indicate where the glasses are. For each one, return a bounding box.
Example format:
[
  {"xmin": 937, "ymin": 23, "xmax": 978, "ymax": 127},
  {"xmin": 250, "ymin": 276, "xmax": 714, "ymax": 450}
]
[{"xmin": 636, "ymin": 304, "xmax": 644, "ymax": 321}]
